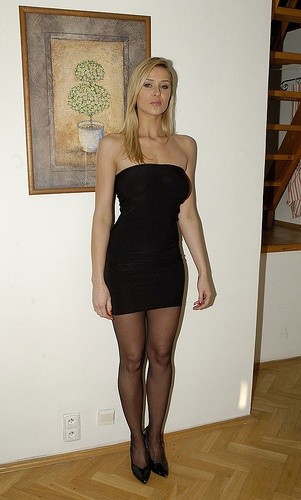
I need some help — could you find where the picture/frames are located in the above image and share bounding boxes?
[18,5,152,195]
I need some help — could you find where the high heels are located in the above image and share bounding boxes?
[144,425,169,478]
[130,440,151,484]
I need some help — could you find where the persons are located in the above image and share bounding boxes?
[90,56,216,484]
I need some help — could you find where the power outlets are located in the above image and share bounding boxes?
[63,412,81,442]
